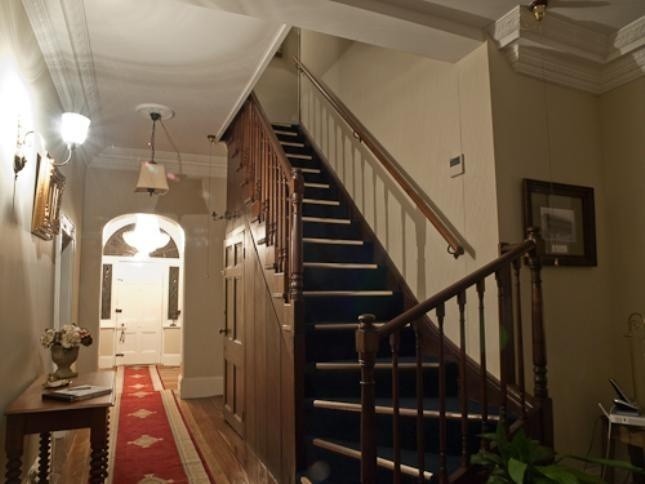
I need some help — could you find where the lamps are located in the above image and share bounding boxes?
[134,113,170,197]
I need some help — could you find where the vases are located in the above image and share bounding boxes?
[52,344,79,379]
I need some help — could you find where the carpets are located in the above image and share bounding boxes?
[106,364,215,484]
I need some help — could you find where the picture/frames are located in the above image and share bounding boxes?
[31,153,67,241]
[523,178,597,266]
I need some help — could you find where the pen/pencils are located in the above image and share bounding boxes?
[68,387,91,391]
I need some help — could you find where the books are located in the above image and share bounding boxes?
[42,384,114,403]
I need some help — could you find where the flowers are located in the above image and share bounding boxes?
[40,323,93,350]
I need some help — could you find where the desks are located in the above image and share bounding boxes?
[3,370,116,483]
